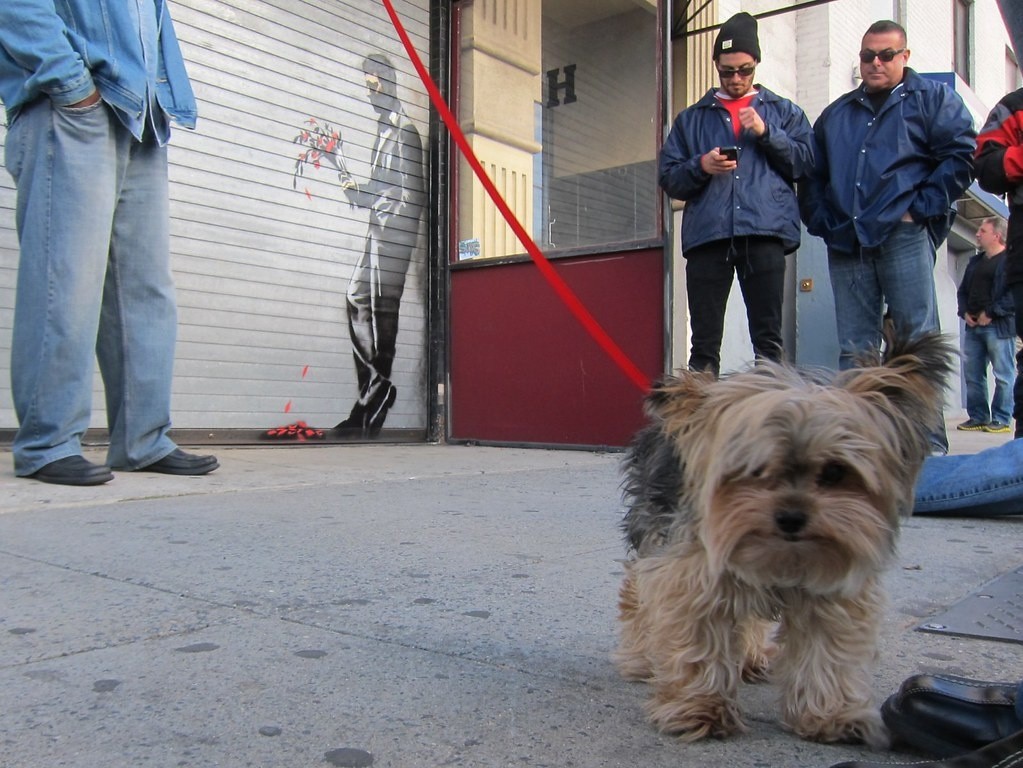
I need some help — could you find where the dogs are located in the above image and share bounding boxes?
[616,317,965,748]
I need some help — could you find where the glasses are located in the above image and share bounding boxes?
[858,49,903,63]
[715,62,754,78]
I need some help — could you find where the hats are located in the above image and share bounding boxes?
[712,12,761,63]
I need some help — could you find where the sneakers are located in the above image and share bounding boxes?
[981,421,1010,432]
[957,418,988,432]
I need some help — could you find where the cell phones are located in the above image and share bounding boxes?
[719,146,738,168]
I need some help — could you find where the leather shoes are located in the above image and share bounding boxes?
[830,731,1023,768]
[879,674,1023,753]
[143,446,219,475]
[29,455,115,484]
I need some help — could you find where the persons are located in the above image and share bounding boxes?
[658,11,819,381]
[974,86,1023,440]
[881,673,1023,758]
[0,0,221,485]
[797,20,978,455]
[957,215,1016,432]
[911,436,1023,516]
